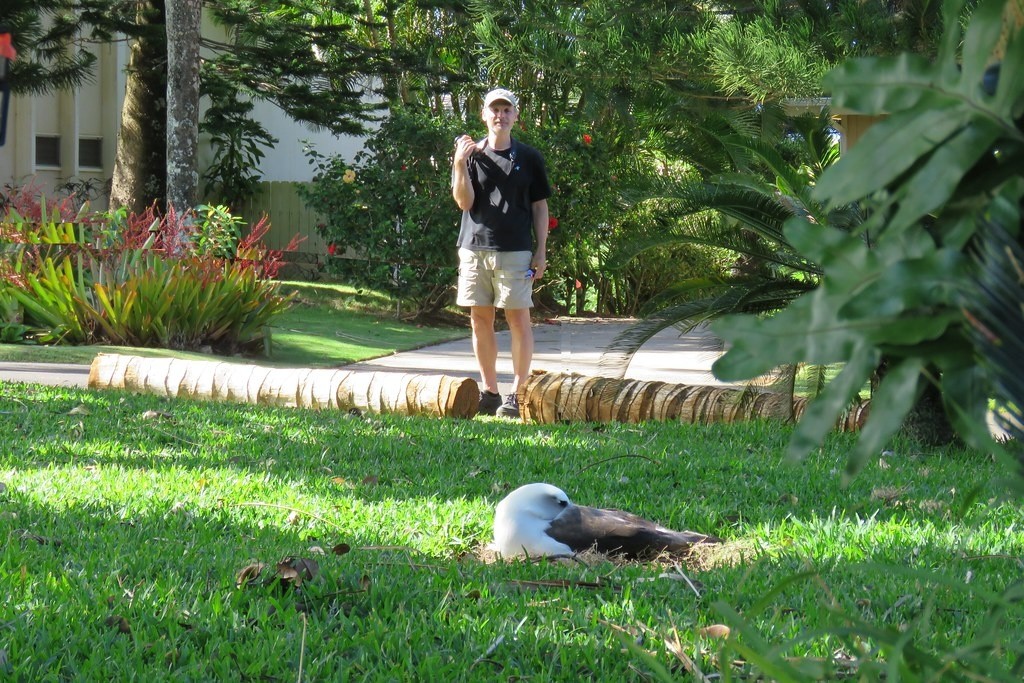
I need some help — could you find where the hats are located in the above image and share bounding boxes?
[484,89,516,109]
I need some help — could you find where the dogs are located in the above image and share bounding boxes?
[488,483,708,559]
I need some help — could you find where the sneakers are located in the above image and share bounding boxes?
[496,392,520,418]
[476,390,502,416]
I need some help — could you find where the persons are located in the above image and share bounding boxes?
[452,89,553,417]
